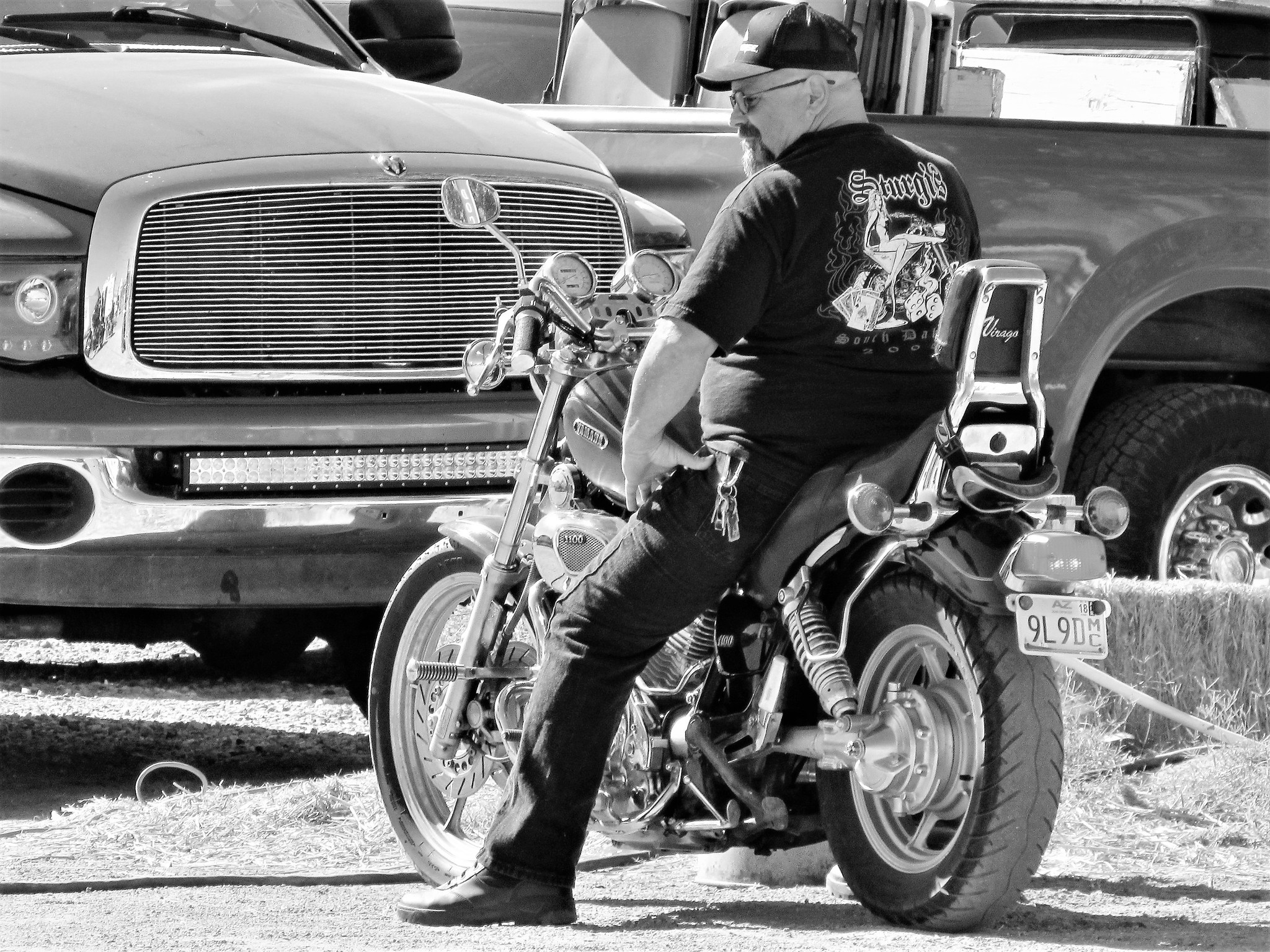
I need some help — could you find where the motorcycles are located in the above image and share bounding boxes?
[364,175,1132,938]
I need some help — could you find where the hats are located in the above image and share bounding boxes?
[695,2,859,91]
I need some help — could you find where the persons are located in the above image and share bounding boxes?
[394,1,982,925]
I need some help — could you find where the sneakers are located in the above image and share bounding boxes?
[398,861,578,927]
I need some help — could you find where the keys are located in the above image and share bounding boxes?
[710,482,740,541]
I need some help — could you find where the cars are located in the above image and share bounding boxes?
[0,0,1270,669]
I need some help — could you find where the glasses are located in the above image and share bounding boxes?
[730,79,835,114]
